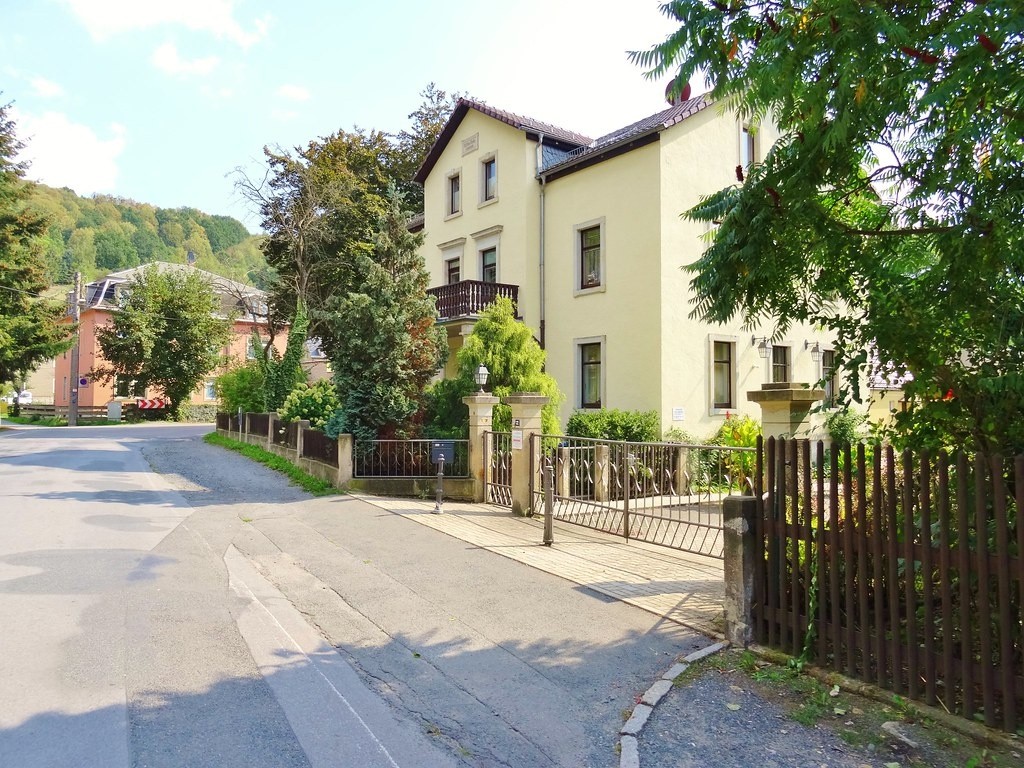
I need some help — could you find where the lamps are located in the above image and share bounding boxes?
[752,335,773,359]
[475,363,491,392]
[805,340,824,362]
